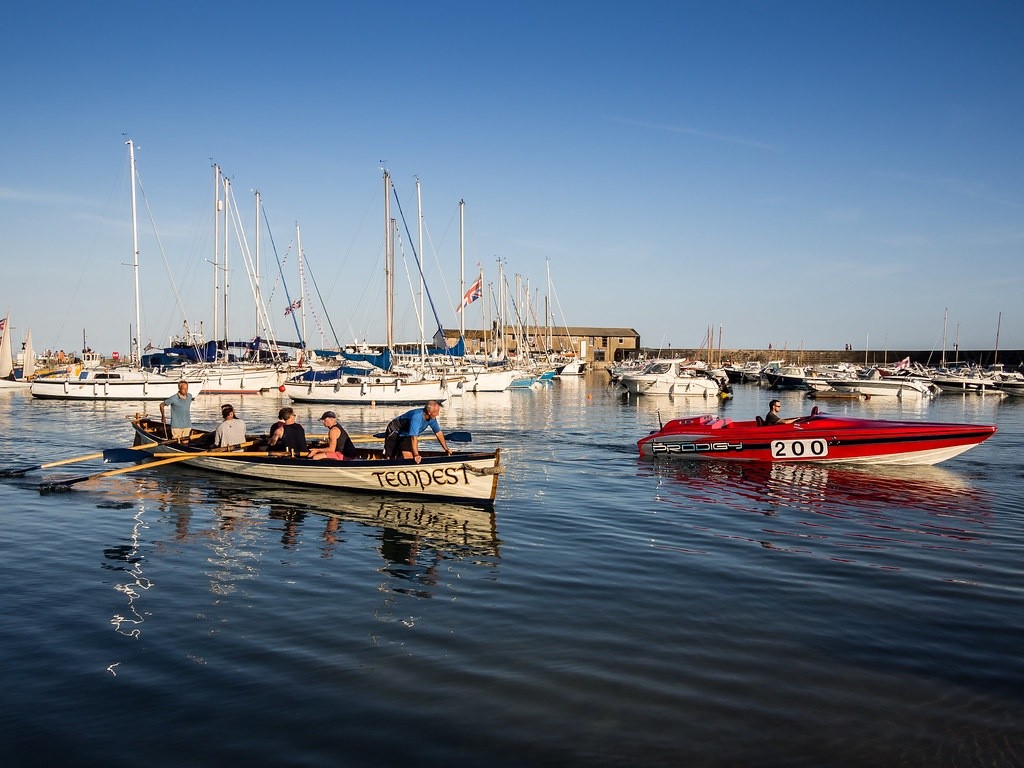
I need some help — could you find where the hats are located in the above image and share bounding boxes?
[320,411,336,421]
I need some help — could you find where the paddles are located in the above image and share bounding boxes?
[102,448,311,463]
[13,431,216,474]
[61,437,270,486]
[263,430,385,438]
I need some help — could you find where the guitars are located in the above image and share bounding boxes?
[351,432,473,443]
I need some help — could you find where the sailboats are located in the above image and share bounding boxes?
[0,160,1024,399]
[31,139,208,401]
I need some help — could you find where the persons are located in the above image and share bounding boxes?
[765,400,801,426]
[308,411,357,460]
[383,402,452,464]
[257,407,309,452]
[160,381,192,445]
[200,404,246,452]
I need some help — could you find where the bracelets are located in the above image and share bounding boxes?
[414,455,420,456]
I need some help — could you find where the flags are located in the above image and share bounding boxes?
[284,300,301,316]
[457,276,482,312]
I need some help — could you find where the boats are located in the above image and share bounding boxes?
[636,412,999,467]
[125,411,503,502]
[638,454,978,506]
[135,455,503,557]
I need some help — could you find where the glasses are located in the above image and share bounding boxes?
[776,406,780,408]
[290,414,296,416]
